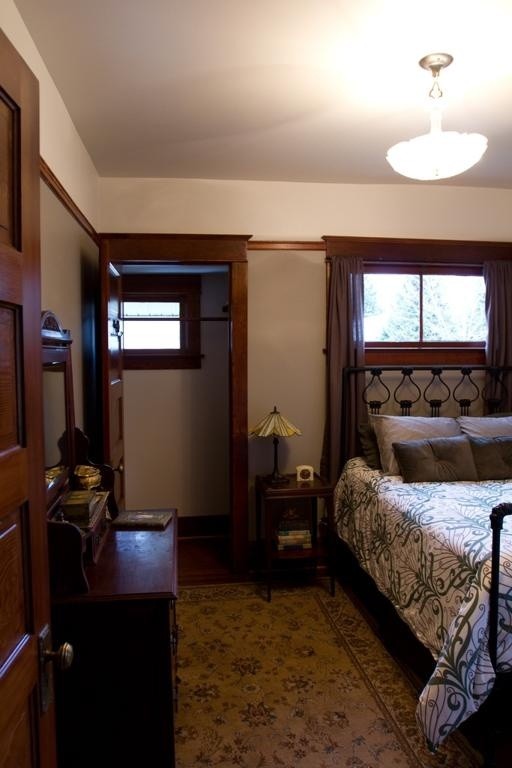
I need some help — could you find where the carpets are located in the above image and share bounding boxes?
[171,577,484,768]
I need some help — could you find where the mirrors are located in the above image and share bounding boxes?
[41,310,75,508]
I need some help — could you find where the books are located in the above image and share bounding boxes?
[113,510,173,531]
[273,521,313,551]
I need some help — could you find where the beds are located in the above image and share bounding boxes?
[335,364,512,756]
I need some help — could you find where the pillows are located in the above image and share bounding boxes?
[457,416,512,437]
[468,434,512,481]
[392,434,478,483]
[368,413,460,477]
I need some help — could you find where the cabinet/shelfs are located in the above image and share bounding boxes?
[50,509,177,768]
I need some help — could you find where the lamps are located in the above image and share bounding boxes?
[249,406,302,483]
[385,52,489,180]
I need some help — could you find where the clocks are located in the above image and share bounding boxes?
[296,464,313,481]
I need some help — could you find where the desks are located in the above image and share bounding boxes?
[257,472,335,602]
